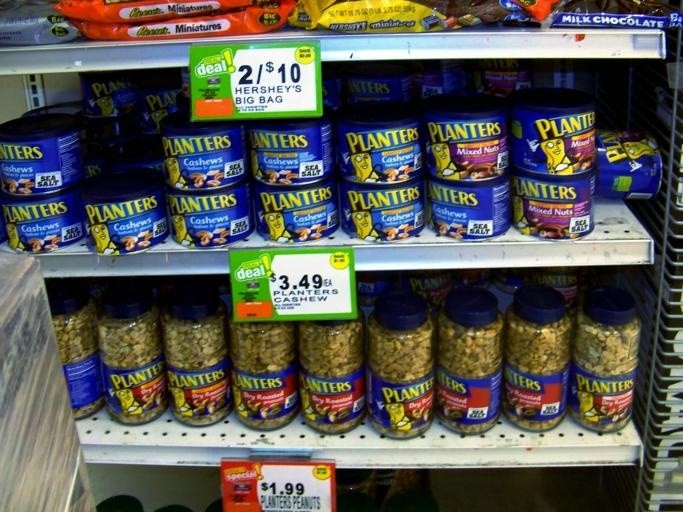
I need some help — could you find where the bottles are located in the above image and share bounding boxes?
[43,286,643,440]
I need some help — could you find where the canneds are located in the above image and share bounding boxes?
[0,60,663,255]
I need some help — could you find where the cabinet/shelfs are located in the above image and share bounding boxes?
[0,28,667,469]
